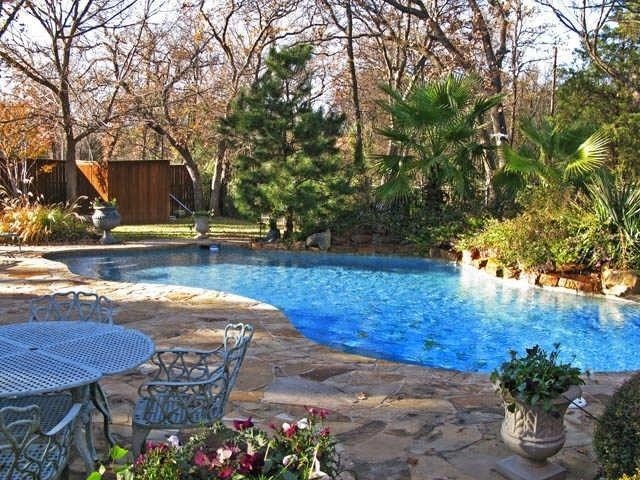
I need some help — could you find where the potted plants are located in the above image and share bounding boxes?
[92,197,122,244]
[191,209,214,239]
[453,216,548,285]
[490,343,598,480]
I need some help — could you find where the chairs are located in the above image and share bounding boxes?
[0,395,82,480]
[29,292,113,460]
[133,323,253,460]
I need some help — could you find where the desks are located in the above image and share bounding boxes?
[0,321,155,475]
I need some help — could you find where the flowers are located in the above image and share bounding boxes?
[86,407,341,480]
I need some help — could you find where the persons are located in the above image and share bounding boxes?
[259,216,293,242]
